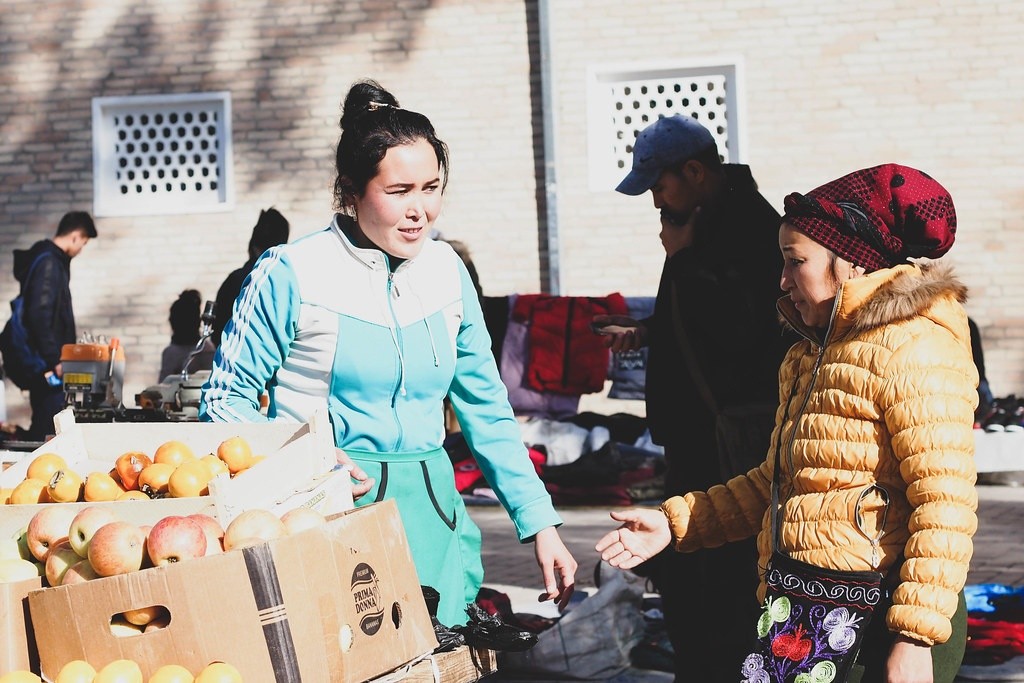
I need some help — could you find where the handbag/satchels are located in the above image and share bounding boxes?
[739,549,884,683]
[0,252,59,389]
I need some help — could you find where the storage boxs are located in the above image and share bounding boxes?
[0,416,498,683]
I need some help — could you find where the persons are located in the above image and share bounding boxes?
[588,116,805,682]
[211,208,290,348]
[159,289,216,383]
[13,211,97,440]
[432,229,482,435]
[197,79,577,642]
[595,162,979,683]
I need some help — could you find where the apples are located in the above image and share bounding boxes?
[0,508,328,683]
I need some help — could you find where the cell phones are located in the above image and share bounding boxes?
[589,322,635,336]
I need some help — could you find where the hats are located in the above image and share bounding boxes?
[782,164,957,271]
[615,116,717,195]
